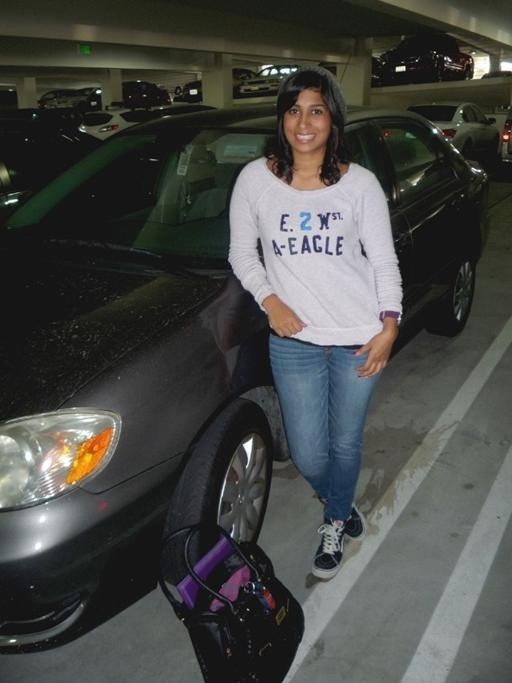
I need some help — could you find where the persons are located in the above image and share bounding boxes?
[228,65,403,579]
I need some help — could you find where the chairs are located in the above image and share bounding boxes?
[185,162,238,224]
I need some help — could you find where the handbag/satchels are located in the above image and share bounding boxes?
[156,521,305,683]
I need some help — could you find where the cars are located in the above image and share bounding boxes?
[38,80,171,110]
[379,36,475,87]
[182,63,312,97]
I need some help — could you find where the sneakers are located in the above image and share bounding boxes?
[311,496,367,579]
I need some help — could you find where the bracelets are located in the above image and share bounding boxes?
[380,311,401,325]
